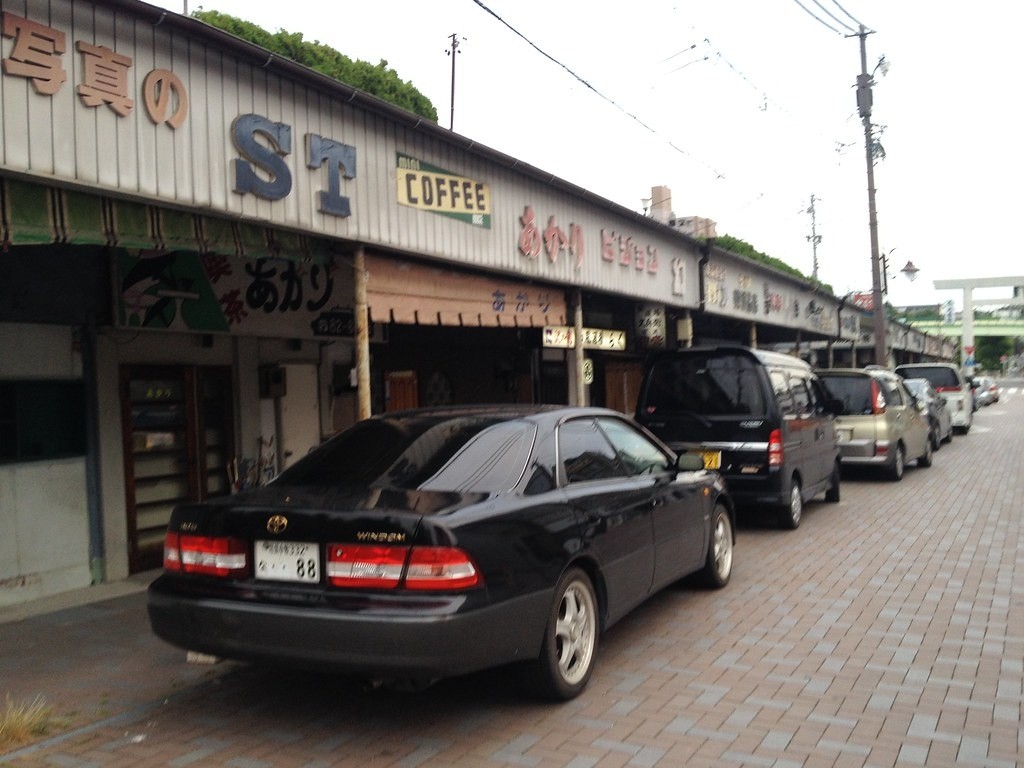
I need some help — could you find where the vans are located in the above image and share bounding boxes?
[634,346,848,529]
[895,364,975,433]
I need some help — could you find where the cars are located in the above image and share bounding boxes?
[904,378,953,450]
[815,365,934,481]
[965,375,998,412]
[147,402,735,702]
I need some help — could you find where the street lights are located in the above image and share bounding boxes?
[871,261,921,369]
